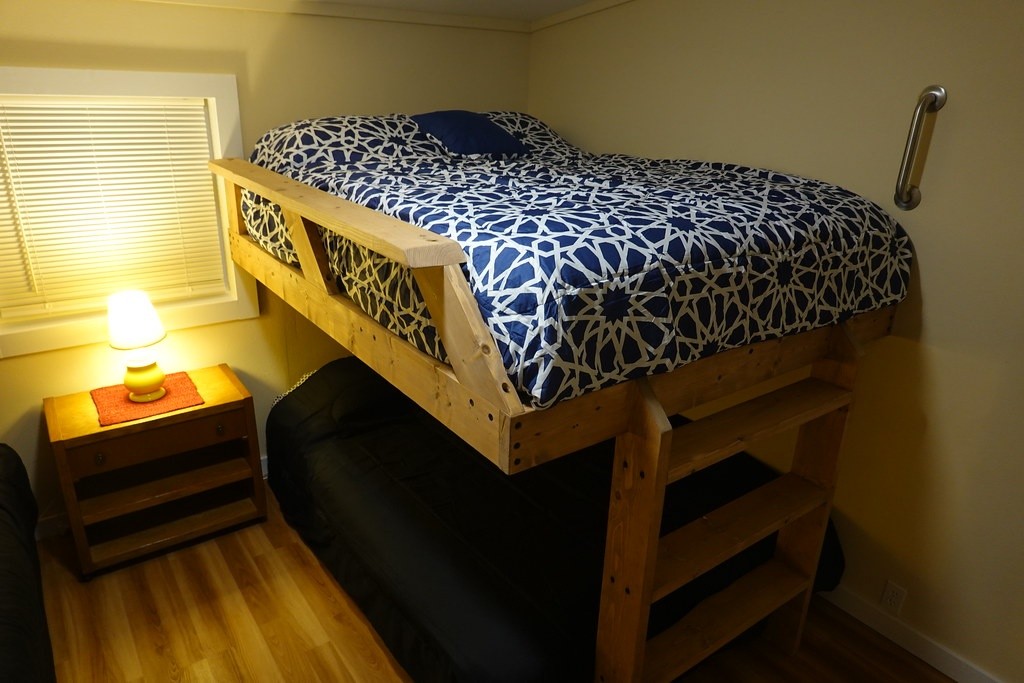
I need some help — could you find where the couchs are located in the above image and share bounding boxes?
[0,442,57,683]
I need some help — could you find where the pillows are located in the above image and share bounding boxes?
[410,110,533,160]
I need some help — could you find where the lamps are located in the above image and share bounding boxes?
[108,288,168,404]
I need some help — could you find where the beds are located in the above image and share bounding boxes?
[208,108,913,682]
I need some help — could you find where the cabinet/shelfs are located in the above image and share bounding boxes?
[43,362,267,582]
